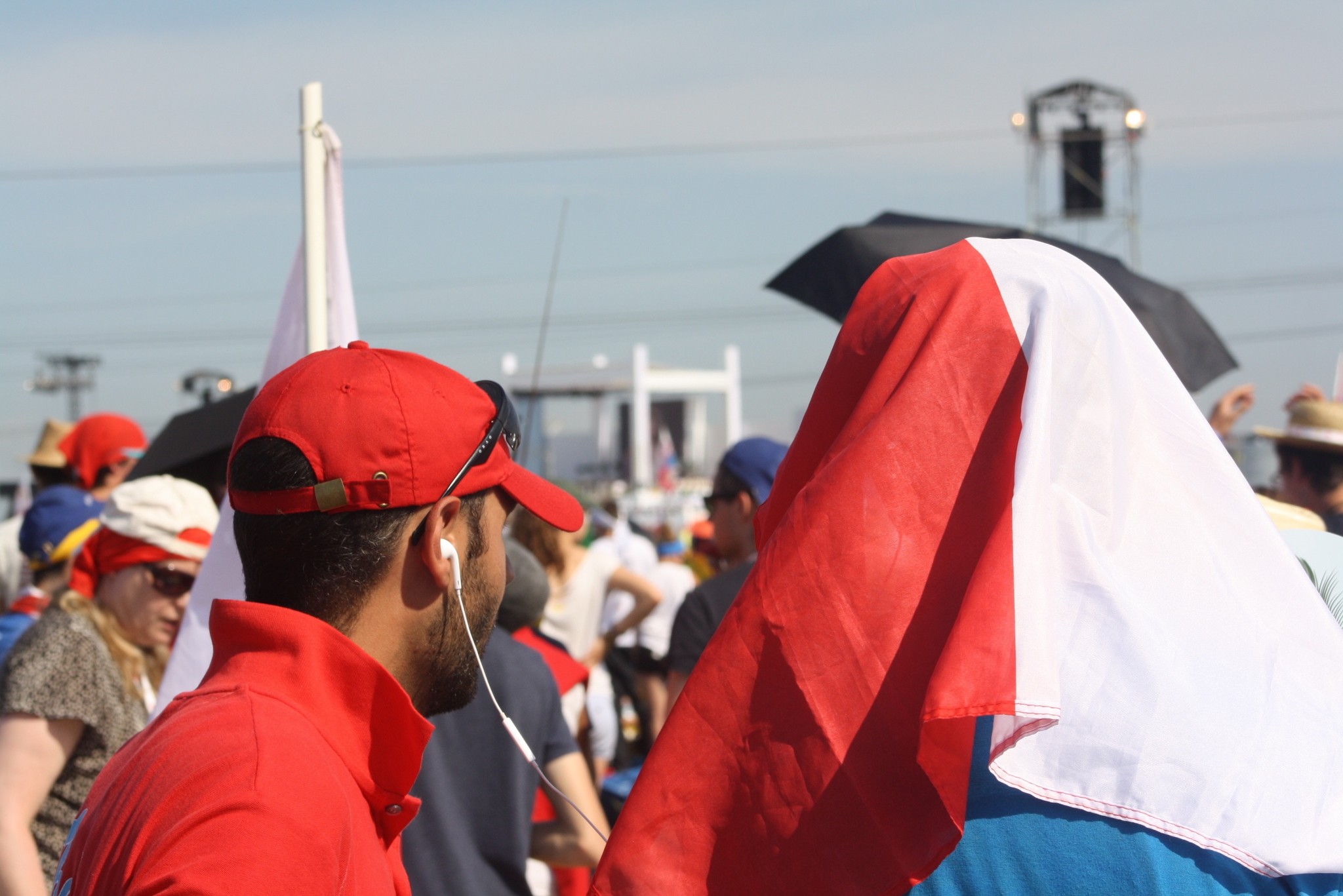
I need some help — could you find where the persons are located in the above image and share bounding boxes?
[0,475,221,896]
[586,236,1343,895]
[53,342,584,896]
[401,627,612,896]
[492,501,720,896]
[0,414,149,672]
[669,436,788,714]
[1208,385,1343,630]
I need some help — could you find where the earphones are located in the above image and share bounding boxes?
[440,538,461,589]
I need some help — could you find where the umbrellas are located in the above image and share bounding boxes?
[767,212,1238,390]
[125,383,257,482]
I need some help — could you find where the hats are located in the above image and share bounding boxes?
[1253,402,1343,454]
[227,341,584,533]
[25,420,73,470]
[723,438,790,505]
[99,472,220,560]
[19,486,109,569]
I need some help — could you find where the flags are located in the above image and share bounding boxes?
[152,128,358,718]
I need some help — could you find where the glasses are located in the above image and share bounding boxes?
[143,563,195,597]
[410,380,521,545]
[1274,439,1343,492]
[705,490,736,515]
